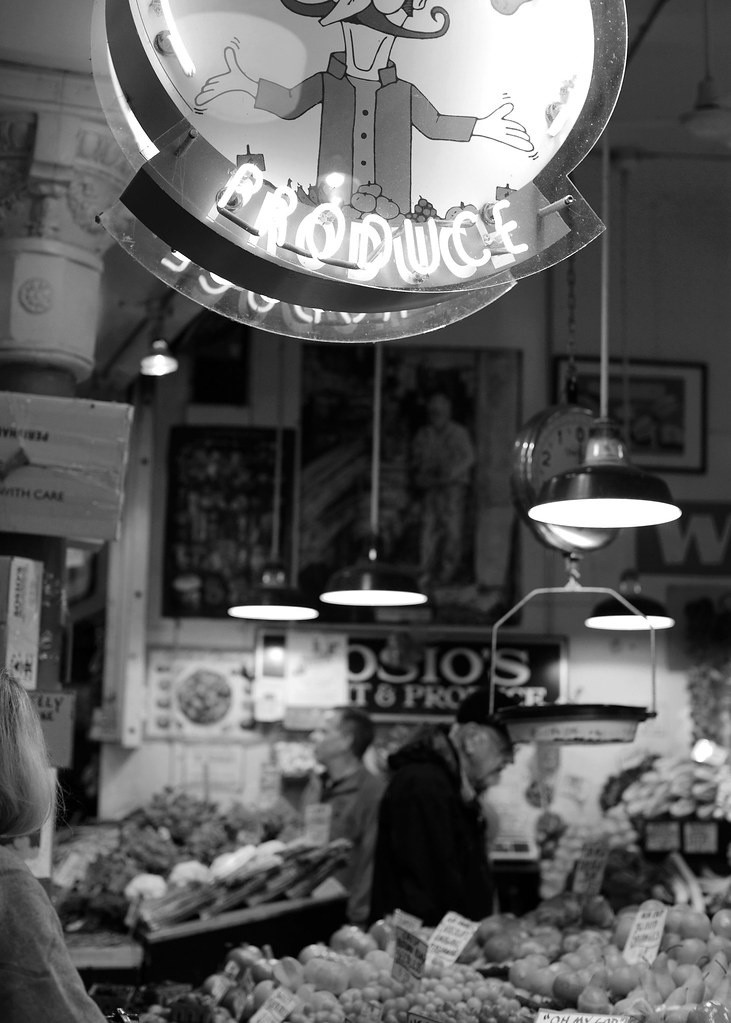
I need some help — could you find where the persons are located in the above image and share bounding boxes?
[369,690,514,930]
[0,672,108,1023]
[311,707,384,932]
[418,397,471,589]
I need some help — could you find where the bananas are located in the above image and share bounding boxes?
[622,753,731,824]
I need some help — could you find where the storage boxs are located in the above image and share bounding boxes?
[251,628,352,722]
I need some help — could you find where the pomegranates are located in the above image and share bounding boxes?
[202,920,400,1023]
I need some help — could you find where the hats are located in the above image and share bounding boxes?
[456,688,519,745]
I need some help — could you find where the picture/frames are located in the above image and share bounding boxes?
[551,353,710,476]
[295,339,525,624]
[161,422,297,619]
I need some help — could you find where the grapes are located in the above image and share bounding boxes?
[293,965,540,1023]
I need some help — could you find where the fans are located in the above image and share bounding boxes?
[679,0,731,137]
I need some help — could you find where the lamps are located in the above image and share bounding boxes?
[315,338,430,611]
[583,569,675,632]
[526,136,684,531]
[225,338,321,622]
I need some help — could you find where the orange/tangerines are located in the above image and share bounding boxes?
[460,898,731,997]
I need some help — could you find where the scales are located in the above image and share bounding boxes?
[489,357,659,745]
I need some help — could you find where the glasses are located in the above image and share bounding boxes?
[485,729,521,757]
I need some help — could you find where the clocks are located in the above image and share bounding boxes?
[510,407,626,555]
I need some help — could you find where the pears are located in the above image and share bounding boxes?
[574,946,731,1023]
